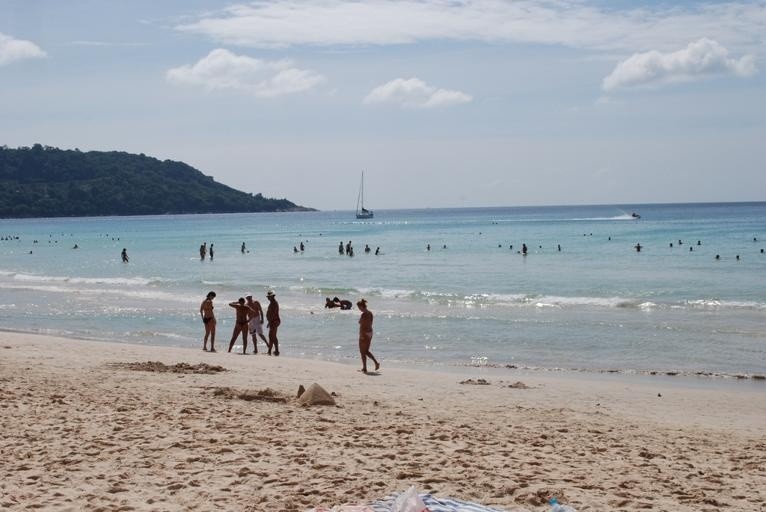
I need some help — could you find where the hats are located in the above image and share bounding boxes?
[266,289,276,297]
[244,291,253,297]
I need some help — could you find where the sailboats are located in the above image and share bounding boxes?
[356,170,373,219]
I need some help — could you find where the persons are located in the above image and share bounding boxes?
[325,297,345,309]
[199,290,218,352]
[120,248,129,263]
[356,299,380,372]
[244,293,269,354]
[523,243,528,254]
[635,243,642,252]
[265,289,281,356]
[195,237,381,263]
[227,297,257,355]
[333,296,353,310]
